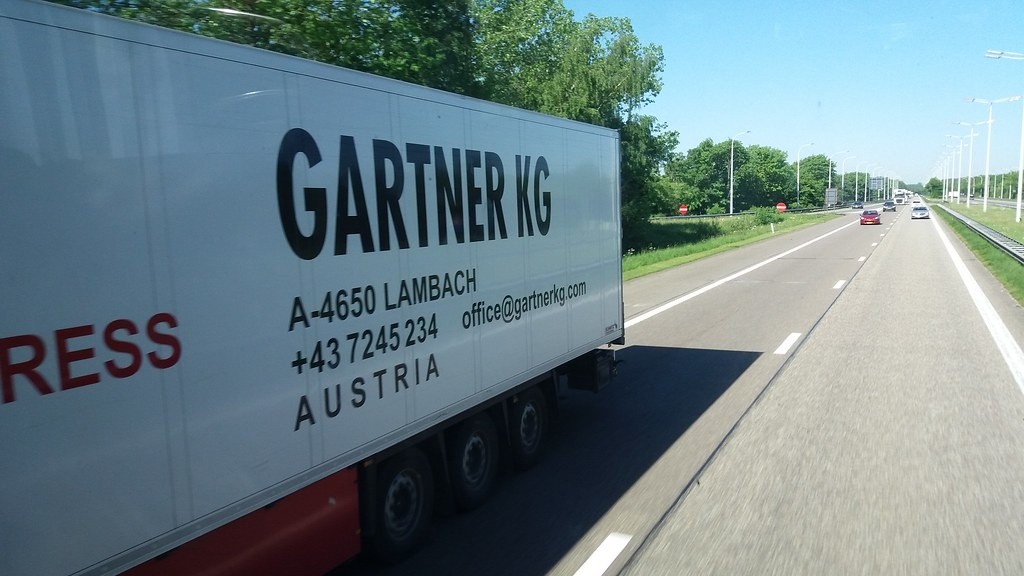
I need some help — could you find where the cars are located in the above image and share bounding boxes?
[852,201,864,209]
[911,206,930,219]
[913,197,921,202]
[860,210,882,224]
[882,202,899,211]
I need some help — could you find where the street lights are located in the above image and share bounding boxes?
[969,96,1021,209]
[730,130,750,216]
[829,150,844,188]
[954,120,992,206]
[797,143,814,207]
[937,132,978,203]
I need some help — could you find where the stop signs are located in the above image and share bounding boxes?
[777,202,787,212]
[679,205,688,215]
